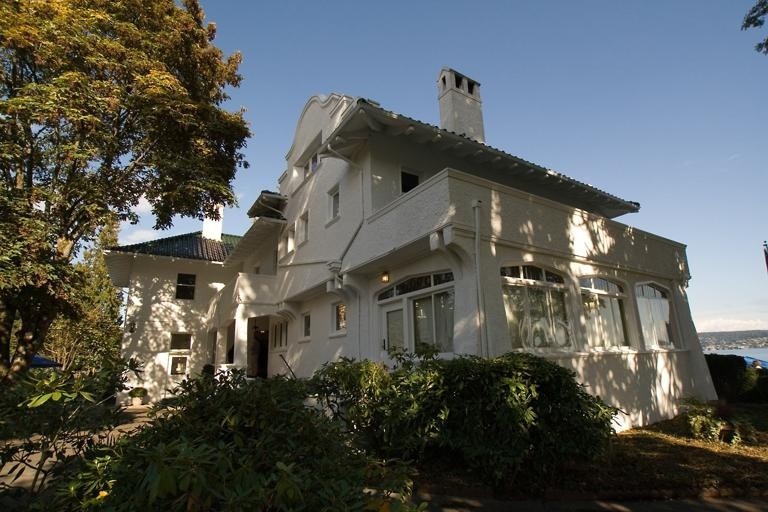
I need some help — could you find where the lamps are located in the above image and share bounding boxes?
[381,270,389,283]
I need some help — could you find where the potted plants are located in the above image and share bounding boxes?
[128,387,148,406]
